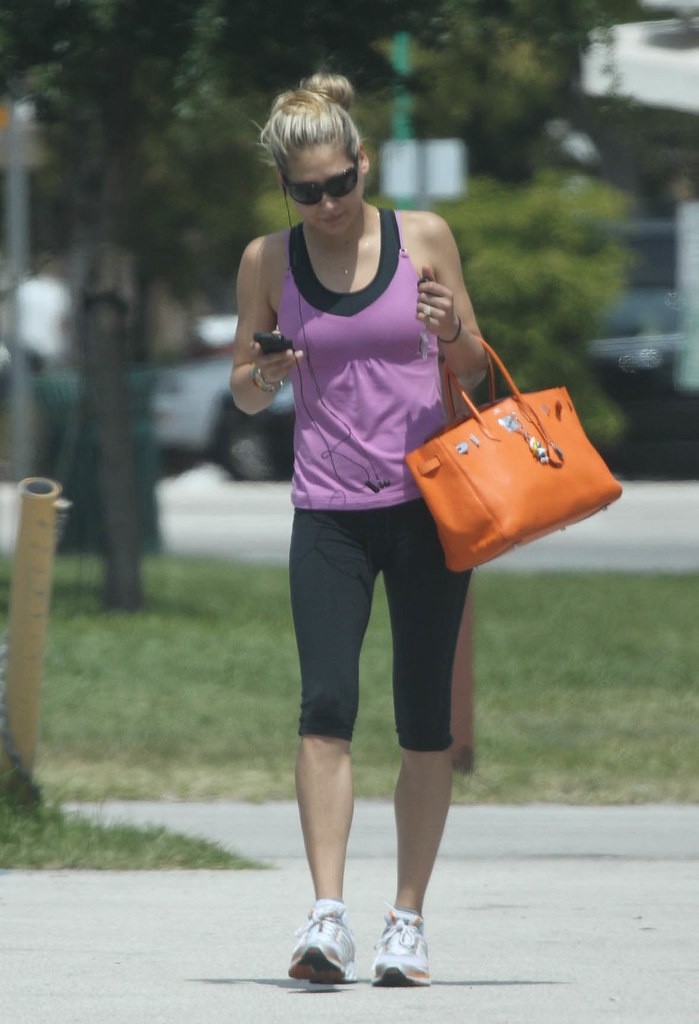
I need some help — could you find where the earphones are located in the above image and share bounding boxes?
[282,181,288,192]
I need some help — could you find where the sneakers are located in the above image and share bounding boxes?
[371,907,432,988]
[287,907,358,983]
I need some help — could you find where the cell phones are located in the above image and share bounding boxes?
[253,334,294,355]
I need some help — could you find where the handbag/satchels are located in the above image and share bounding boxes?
[401,338,623,572]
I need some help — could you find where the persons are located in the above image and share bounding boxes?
[230,71,490,989]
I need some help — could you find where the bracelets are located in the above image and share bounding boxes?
[251,363,287,394]
[437,316,461,344]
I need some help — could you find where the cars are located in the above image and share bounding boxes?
[150,312,298,483]
[585,332,699,482]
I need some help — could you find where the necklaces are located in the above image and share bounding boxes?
[306,229,373,274]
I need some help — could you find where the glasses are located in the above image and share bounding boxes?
[284,156,363,209]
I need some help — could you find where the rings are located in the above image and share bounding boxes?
[424,304,431,319]
